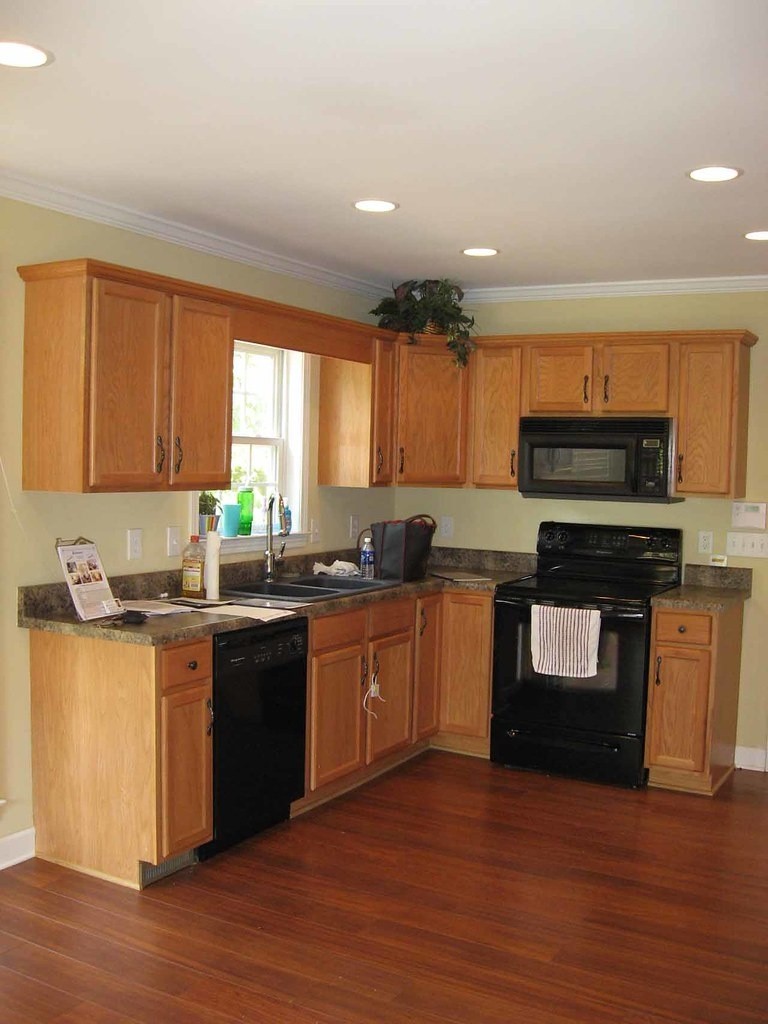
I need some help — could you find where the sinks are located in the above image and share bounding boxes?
[224,584,339,598]
[287,576,387,590]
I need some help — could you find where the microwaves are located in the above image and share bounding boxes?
[518,413,686,505]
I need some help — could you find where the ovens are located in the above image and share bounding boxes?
[489,600,652,792]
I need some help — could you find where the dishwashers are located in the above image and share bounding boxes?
[195,616,309,863]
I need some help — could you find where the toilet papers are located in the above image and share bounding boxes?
[203,530,222,600]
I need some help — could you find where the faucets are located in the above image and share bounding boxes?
[264,492,288,584]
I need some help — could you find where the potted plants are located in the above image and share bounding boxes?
[198,489,223,539]
[368,277,480,369]
[232,463,268,536]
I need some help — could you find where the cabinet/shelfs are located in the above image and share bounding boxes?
[305,594,411,808]
[644,605,744,800]
[523,331,680,417]
[28,629,212,890]
[411,588,438,758]
[437,586,496,760]
[316,324,400,490]
[16,257,235,495]
[395,332,468,489]
[675,329,759,500]
[468,335,525,491]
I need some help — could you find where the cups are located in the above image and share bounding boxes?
[222,504,241,537]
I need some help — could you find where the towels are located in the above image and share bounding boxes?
[312,558,363,578]
[530,604,602,679]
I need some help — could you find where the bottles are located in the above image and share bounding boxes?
[361,538,374,580]
[236,486,254,535]
[181,535,206,600]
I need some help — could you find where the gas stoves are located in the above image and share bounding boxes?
[494,521,683,611]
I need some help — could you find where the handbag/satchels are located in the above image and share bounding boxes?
[357,512,437,582]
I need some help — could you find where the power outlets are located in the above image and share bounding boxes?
[697,529,713,555]
[127,528,144,561]
[439,515,453,539]
[310,518,322,544]
[349,513,360,539]
